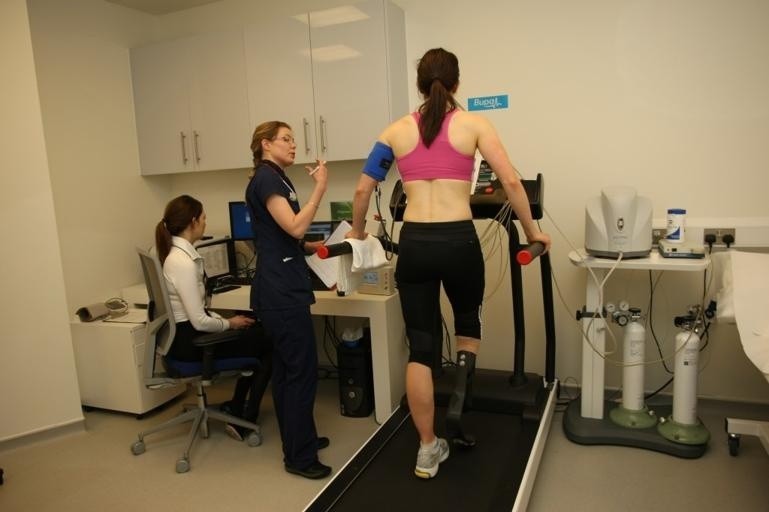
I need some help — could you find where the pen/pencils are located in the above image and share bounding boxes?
[309,160,327,176]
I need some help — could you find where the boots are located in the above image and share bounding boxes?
[444,351,478,446]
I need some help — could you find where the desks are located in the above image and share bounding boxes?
[123,279,411,425]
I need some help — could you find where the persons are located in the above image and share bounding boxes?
[155,195,272,439]
[345,48,550,480]
[244,121,332,477]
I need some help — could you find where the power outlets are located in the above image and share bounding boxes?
[704,228,735,245]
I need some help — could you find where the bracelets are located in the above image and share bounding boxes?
[308,198,321,209]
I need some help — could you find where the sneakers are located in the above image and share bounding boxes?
[415,437,449,478]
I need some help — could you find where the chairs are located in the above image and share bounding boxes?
[131,246,263,473]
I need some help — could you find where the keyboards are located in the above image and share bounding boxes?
[223,277,250,285]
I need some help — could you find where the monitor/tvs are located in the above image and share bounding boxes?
[227,200,261,241]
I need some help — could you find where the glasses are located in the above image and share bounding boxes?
[273,133,296,144]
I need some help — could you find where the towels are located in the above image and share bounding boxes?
[336,234,389,295]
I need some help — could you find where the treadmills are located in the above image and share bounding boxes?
[303,173,562,512]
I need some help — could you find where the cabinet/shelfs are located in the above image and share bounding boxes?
[68,309,187,419]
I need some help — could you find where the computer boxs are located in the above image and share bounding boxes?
[335,326,375,418]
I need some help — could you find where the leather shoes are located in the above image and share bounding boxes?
[318,436,329,450]
[285,459,331,480]
[221,399,257,440]
